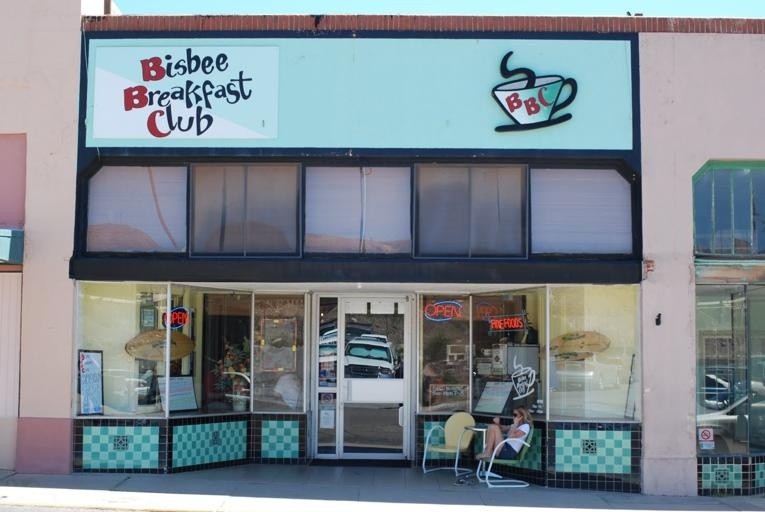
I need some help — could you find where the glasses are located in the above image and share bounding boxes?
[512,413,517,417]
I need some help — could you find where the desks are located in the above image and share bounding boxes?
[464,425,491,481]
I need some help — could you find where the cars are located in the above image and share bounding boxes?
[320,329,398,378]
[555,351,637,392]
[98,366,151,408]
[696,363,765,459]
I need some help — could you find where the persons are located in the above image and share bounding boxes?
[476,406,532,459]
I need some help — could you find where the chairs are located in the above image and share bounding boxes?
[422,412,477,477]
[476,422,534,488]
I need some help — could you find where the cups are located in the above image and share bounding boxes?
[480,348,492,357]
[491,75,577,124]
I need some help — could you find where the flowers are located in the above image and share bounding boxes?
[209,336,251,403]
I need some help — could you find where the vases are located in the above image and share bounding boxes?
[233,375,247,412]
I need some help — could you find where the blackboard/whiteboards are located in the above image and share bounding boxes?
[157,375,199,412]
[474,381,514,414]
[78,348,103,416]
